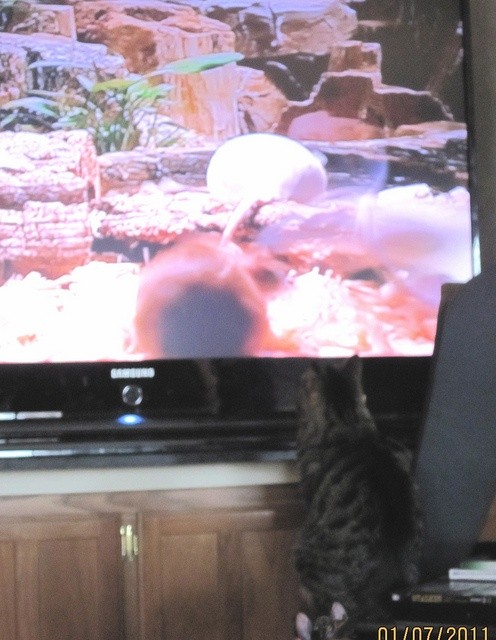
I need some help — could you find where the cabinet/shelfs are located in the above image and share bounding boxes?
[142,487,312,640]
[1,496,141,640]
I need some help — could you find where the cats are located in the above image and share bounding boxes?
[280,352,426,618]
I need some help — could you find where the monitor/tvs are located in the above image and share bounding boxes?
[0,3,484,473]
